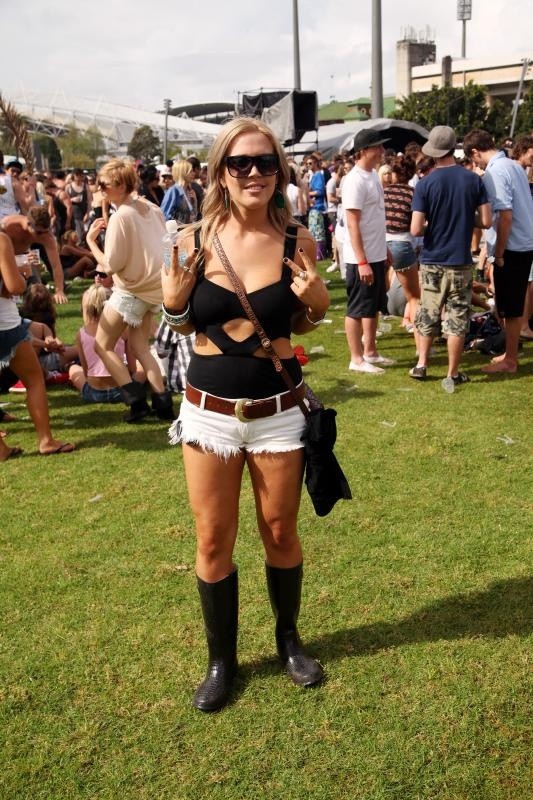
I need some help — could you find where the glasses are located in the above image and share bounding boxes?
[93,270,108,278]
[98,180,112,189]
[306,162,314,165]
[32,226,49,235]
[224,154,278,177]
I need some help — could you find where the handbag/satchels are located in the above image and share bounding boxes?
[299,408,352,516]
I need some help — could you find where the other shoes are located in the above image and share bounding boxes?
[47,371,69,384]
[8,381,27,391]
[326,264,339,274]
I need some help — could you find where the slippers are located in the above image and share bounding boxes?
[10,447,21,457]
[56,442,71,453]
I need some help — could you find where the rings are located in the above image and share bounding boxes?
[183,265,190,272]
[298,270,307,279]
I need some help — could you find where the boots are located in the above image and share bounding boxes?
[193,564,238,711]
[264,557,323,685]
[119,378,150,422]
[150,391,174,418]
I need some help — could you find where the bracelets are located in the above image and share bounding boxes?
[306,311,326,326]
[358,260,368,265]
[161,302,191,326]
[495,257,504,258]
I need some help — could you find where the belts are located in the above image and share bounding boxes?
[185,380,305,422]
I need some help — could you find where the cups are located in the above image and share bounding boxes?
[15,254,27,266]
[30,249,40,266]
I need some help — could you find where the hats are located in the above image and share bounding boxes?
[422,126,456,157]
[354,129,392,151]
[161,170,173,178]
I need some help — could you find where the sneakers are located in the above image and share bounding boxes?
[448,372,471,385]
[366,355,394,364]
[350,360,384,374]
[409,365,426,379]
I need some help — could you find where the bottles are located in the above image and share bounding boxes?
[162,220,188,275]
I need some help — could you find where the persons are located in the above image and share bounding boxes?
[0,126,533,461]
[86,157,177,424]
[160,114,330,713]
[341,128,398,373]
